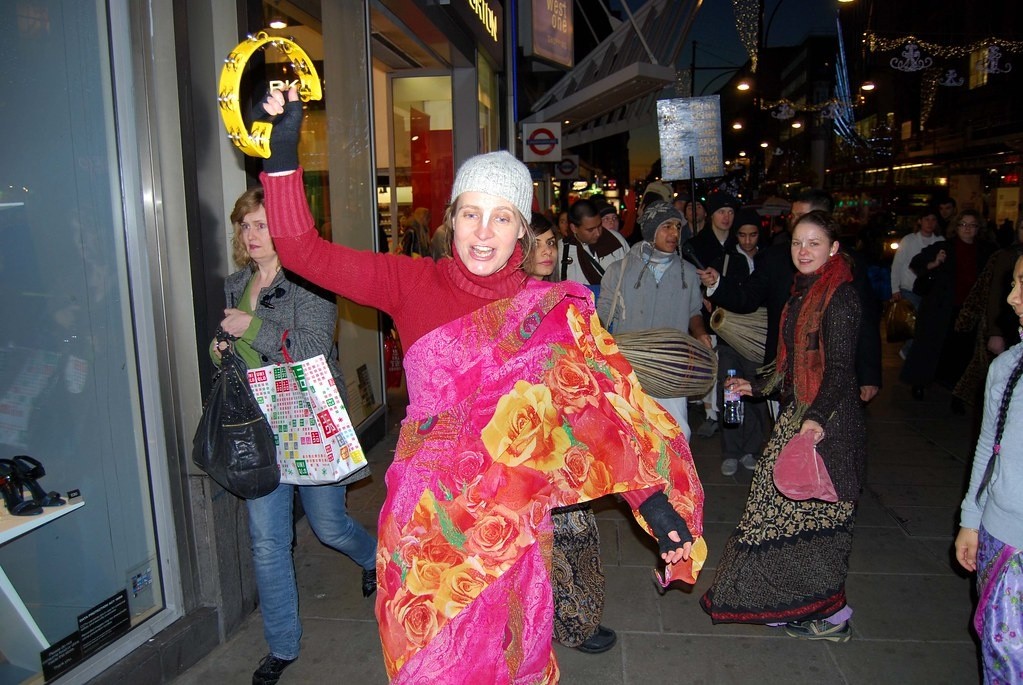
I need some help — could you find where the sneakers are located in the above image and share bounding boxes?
[361,569,377,600]
[784,617,853,644]
[252,653,299,684]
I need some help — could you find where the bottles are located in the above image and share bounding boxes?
[724,369,741,423]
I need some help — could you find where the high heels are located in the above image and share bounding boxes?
[0,455,67,516]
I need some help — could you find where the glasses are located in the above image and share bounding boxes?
[959,221,979,229]
[601,215,619,222]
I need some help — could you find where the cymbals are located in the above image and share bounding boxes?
[217,31,323,159]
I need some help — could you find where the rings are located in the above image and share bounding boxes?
[815,435,823,437]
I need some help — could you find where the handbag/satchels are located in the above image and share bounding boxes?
[190,320,282,499]
[243,335,369,486]
[884,298,919,344]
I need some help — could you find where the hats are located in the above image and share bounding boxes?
[634,200,689,291]
[590,199,617,216]
[707,191,736,215]
[734,207,762,230]
[641,181,675,204]
[450,149,534,225]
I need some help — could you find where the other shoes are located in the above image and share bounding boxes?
[721,454,757,476]
[912,383,924,401]
[698,417,719,437]
[576,624,616,654]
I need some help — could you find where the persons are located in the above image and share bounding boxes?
[955,253,1023,685]
[520,191,792,654]
[890,196,1023,408]
[699,211,866,643]
[378,207,453,339]
[209,187,377,685]
[252,80,692,685]
[696,189,882,511]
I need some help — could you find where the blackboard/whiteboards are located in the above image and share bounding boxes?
[656,95,725,181]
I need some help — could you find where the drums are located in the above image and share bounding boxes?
[611,328,718,399]
[709,306,768,364]
